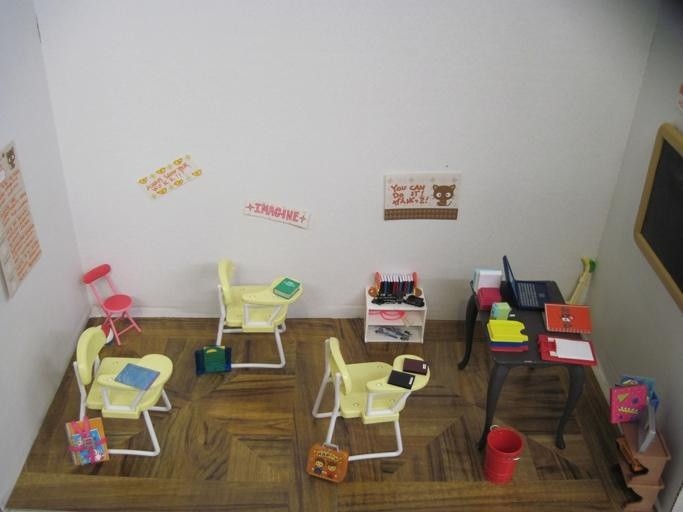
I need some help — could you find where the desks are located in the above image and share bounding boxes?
[241,276,303,332]
[361,354,430,424]
[97,354,173,419]
[457,280,586,454]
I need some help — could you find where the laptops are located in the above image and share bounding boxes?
[503,255,551,312]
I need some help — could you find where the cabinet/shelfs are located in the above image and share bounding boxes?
[364,287,427,344]
[616,421,672,512]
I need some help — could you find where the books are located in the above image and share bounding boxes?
[65,417,110,467]
[273,278,300,300]
[378,272,414,295]
[542,303,592,334]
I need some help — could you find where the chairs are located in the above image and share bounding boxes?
[82,264,142,346]
[72,327,173,457]
[215,257,303,369]
[312,337,430,462]
